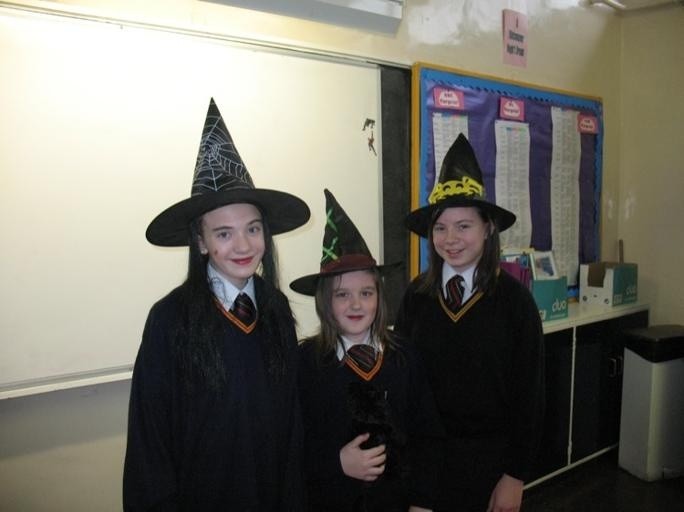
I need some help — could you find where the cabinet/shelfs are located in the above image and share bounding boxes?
[517,296,650,490]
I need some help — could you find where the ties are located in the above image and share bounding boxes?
[349,343,377,373]
[235,294,252,323]
[446,274,465,315]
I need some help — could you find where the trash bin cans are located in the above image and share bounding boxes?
[616,323,684,483]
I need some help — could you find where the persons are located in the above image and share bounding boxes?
[294,189,436,511]
[123,96,313,512]
[391,132,547,511]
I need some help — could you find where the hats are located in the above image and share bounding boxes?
[145,97,311,248]
[404,133,516,238]
[289,189,403,296]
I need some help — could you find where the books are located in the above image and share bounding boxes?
[498,245,558,291]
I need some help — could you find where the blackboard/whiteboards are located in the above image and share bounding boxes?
[0,0,387,402]
